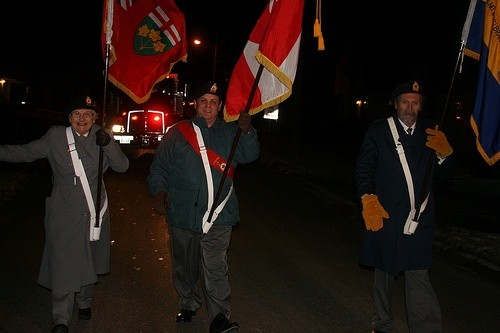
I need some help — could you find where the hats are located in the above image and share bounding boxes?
[69,95,97,115]
[194,79,221,100]
[390,79,427,100]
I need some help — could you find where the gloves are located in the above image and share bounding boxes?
[152,192,167,217]
[95,129,112,147]
[237,109,252,130]
[361,194,389,231]
[425,128,453,159]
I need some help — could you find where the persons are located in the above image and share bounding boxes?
[148,82,260,333]
[356,81,457,333]
[0,95,129,333]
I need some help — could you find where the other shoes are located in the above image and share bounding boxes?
[176,309,193,322]
[51,324,68,333]
[209,313,241,333]
[79,307,92,321]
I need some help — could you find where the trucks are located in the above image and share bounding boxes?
[107,74,198,146]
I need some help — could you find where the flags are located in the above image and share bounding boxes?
[101,0,189,103]
[459,0,500,166]
[222,0,325,122]
